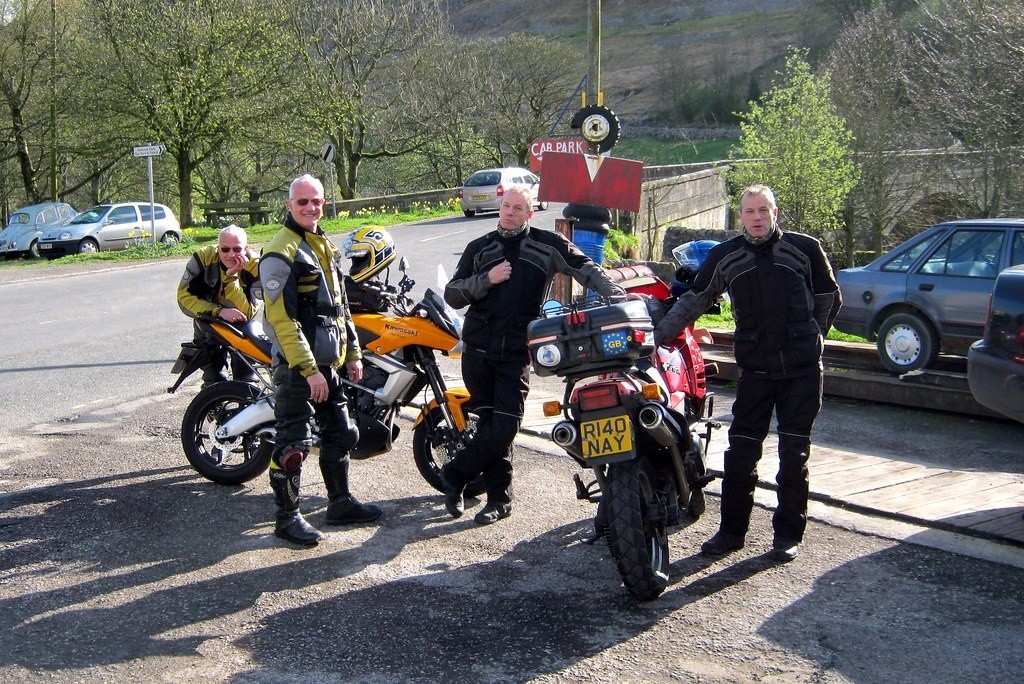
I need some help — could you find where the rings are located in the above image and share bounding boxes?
[316,391,318,393]
[241,265,244,267]
[233,317,235,320]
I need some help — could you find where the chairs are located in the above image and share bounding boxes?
[488,175,498,182]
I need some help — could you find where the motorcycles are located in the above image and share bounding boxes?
[525,273,724,601]
[165,225,516,498]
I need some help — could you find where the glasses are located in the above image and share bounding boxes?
[291,199,324,206]
[219,246,244,252]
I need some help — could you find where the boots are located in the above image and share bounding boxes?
[268,468,324,544]
[319,456,383,523]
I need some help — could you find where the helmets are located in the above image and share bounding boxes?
[344,226,396,283]
[672,240,722,296]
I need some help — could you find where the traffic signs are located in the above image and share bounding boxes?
[133,145,163,157]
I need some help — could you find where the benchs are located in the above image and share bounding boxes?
[919,261,997,278]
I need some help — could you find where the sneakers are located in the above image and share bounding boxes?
[439,466,463,517]
[701,531,745,554]
[773,536,799,559]
[475,505,509,523]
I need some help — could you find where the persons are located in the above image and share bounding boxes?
[177,224,262,452]
[440,185,628,524]
[258,174,382,545]
[655,185,842,562]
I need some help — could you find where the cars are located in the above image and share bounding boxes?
[458,166,550,219]
[966,262,1023,425]
[0,201,100,259]
[832,215,1024,377]
[35,201,183,261]
